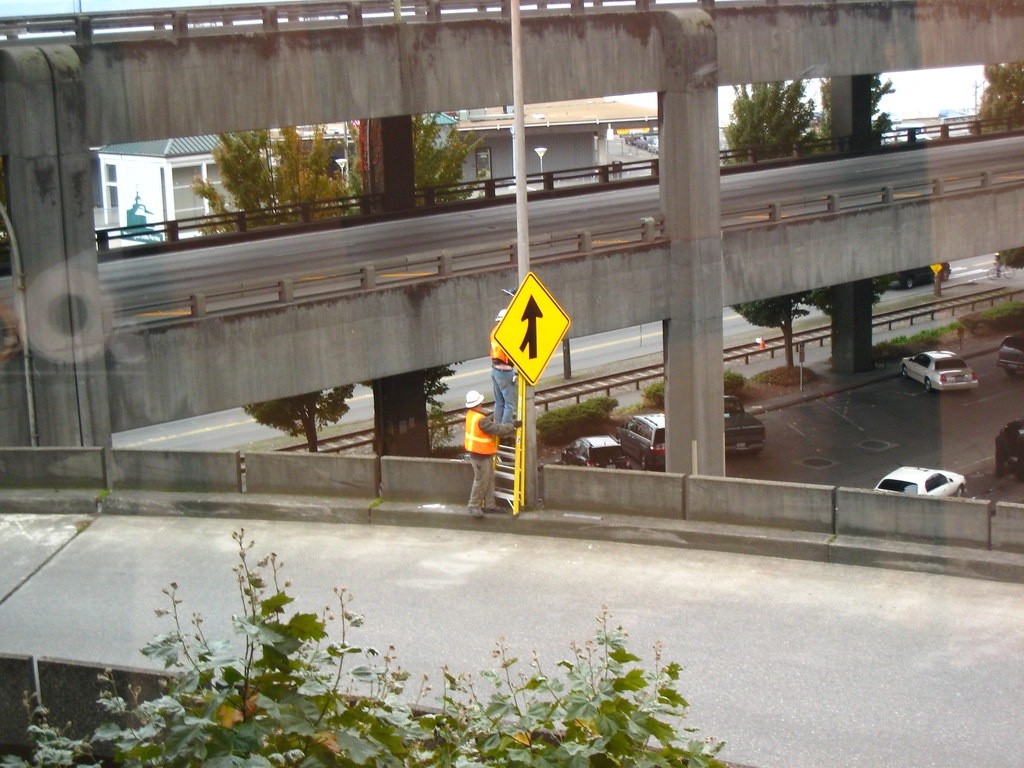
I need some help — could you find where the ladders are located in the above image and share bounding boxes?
[478,347,525,520]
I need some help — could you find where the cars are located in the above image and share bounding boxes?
[901,350,977,393]
[873,465,969,499]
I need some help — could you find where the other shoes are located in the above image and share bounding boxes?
[468,505,484,518]
[481,506,508,513]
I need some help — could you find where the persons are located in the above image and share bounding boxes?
[490,309,517,438]
[464,390,515,517]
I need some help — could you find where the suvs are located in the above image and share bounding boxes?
[562,433,632,471]
[995,333,1024,376]
[724,397,766,458]
[994,421,1024,481]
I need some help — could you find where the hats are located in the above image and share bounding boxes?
[465,390,484,408]
[495,309,506,321]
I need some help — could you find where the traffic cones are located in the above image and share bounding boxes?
[759,336,766,349]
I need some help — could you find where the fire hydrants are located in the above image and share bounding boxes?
[993,252,1003,277]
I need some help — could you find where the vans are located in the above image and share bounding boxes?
[895,262,951,289]
[615,413,665,471]
